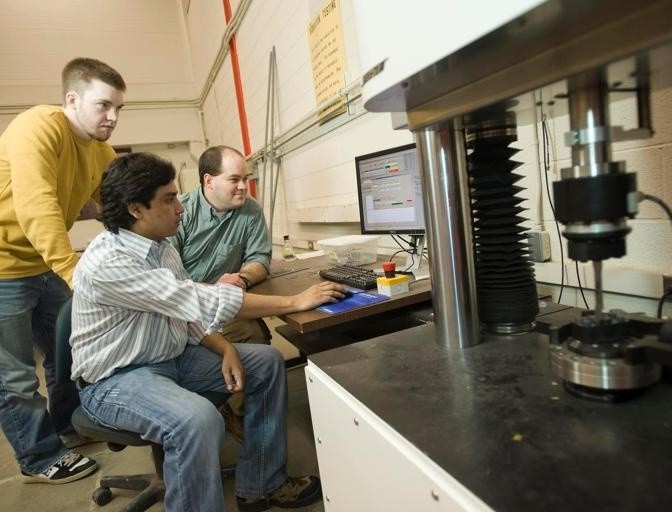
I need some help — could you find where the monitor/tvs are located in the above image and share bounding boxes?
[354,143,431,284]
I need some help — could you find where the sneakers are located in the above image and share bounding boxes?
[235,475,320,512]
[21,451,97,485]
[60,431,105,448]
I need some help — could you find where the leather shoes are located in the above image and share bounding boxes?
[220,404,244,444]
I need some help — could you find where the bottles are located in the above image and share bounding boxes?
[283,235,296,261]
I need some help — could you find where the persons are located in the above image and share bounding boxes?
[0,56,127,485]
[68,151,348,512]
[165,142,274,446]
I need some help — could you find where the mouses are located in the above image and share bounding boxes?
[319,290,354,305]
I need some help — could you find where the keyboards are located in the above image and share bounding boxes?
[319,263,383,291]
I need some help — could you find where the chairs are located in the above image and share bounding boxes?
[53,296,237,512]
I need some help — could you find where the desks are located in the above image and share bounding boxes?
[249,241,433,335]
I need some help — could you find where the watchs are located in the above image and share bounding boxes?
[239,275,252,290]
